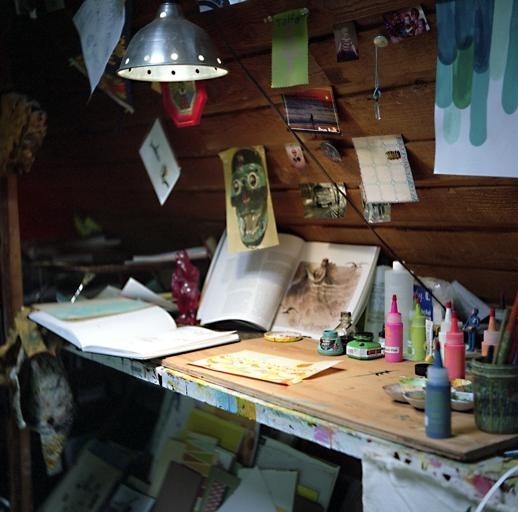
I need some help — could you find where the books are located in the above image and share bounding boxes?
[354,133,418,207]
[39,390,340,512]
[198,225,380,342]
[27,302,241,360]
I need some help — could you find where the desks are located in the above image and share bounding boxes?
[62,297,515,505]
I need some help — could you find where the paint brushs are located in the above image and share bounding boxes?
[70,272,94,303]
[492,300,516,366]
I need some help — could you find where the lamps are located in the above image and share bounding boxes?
[116,3,230,84]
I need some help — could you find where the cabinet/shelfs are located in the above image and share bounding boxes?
[1,161,36,512]
